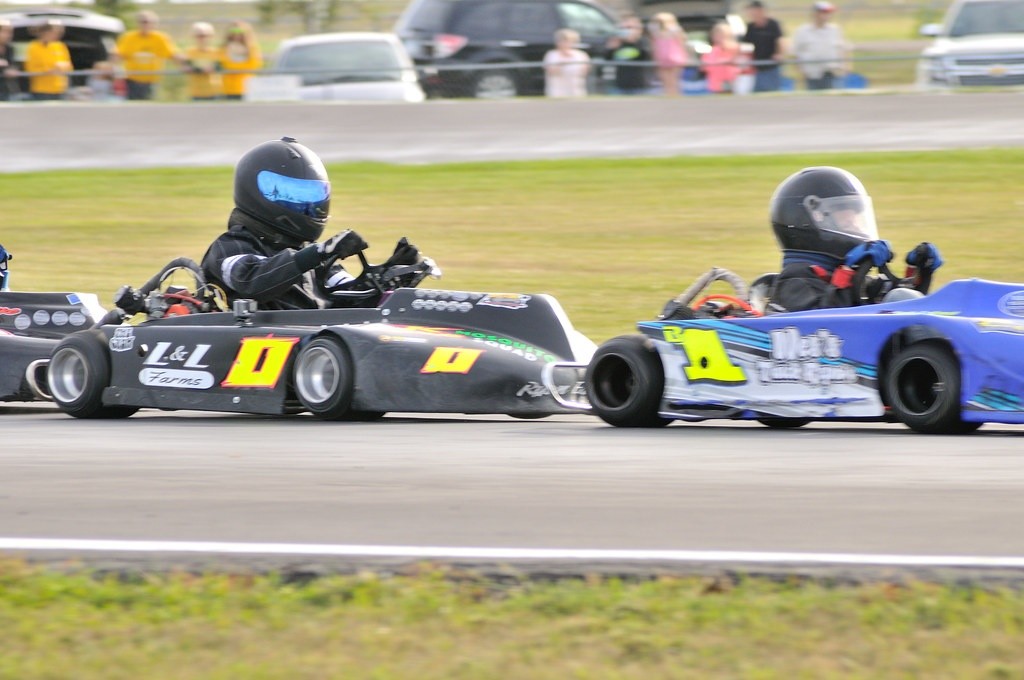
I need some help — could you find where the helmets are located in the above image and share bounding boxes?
[771,166,879,261]
[232,135,330,240]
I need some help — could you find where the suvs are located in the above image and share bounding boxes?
[910,0,1024,86]
[396,0,648,99]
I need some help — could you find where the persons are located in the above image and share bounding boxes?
[744,0,788,92]
[544,28,590,98]
[791,2,841,90]
[0,22,15,102]
[764,167,943,314]
[25,24,72,100]
[114,11,263,100]
[700,21,752,93]
[601,13,687,95]
[201,137,433,312]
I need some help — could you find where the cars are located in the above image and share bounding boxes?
[630,0,731,47]
[276,26,429,105]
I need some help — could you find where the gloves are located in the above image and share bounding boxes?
[905,242,942,272]
[844,240,892,269]
[381,237,420,290]
[315,227,369,265]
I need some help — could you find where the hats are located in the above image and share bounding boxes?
[193,22,215,37]
[813,2,835,13]
